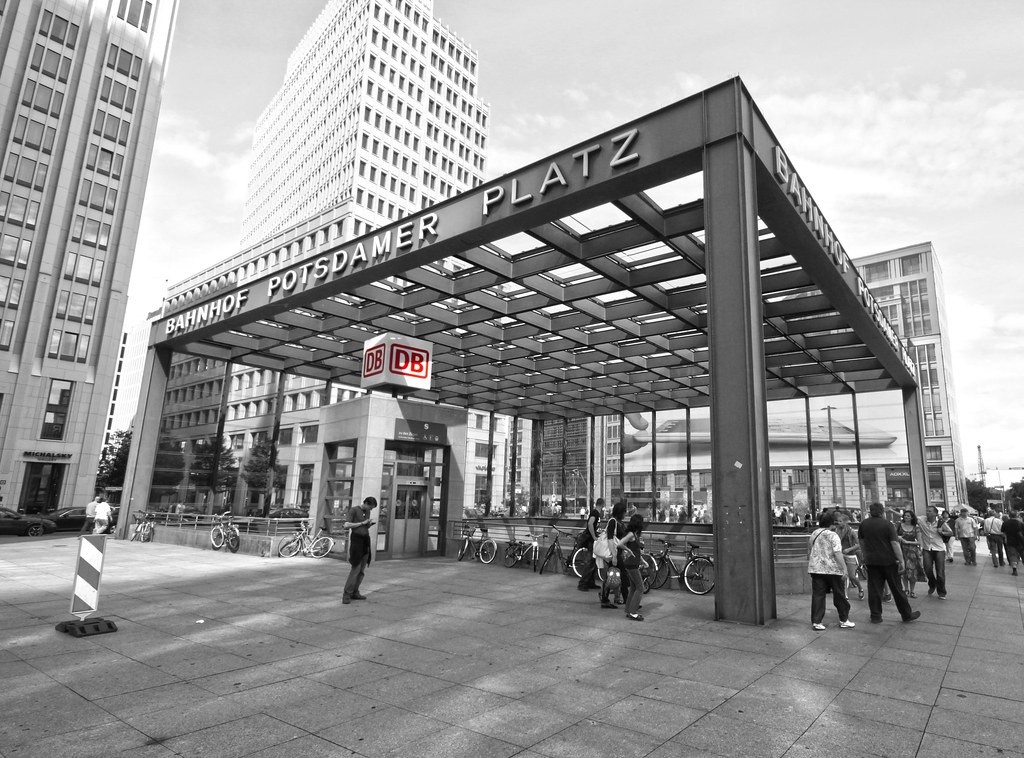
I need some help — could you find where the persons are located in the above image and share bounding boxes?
[896,509,924,599]
[772,504,906,526]
[807,513,856,630]
[886,507,953,600]
[836,515,865,601]
[858,503,922,624]
[882,579,892,601]
[580,503,712,521]
[940,505,1024,575]
[167,500,186,515]
[578,497,606,592]
[342,496,377,604]
[599,502,646,622]
[77,496,113,533]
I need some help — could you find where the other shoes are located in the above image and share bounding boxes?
[614,599,623,604]
[904,611,920,621]
[1000,561,1006,566]
[601,604,617,608]
[844,585,946,603]
[994,566,998,567]
[626,612,644,620]
[589,584,601,589]
[580,588,589,591]
[965,562,971,565]
[871,617,883,623]
[972,562,976,565]
[1012,568,1018,576]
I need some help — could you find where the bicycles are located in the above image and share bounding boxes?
[458,521,498,564]
[850,560,868,588]
[278,520,335,558]
[642,539,715,596]
[131,509,156,543]
[504,533,548,572]
[210,511,241,549]
[539,523,659,594]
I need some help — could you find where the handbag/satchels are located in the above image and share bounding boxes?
[577,533,591,549]
[937,518,950,542]
[593,518,617,563]
[987,533,991,539]
[596,556,606,568]
[605,567,621,589]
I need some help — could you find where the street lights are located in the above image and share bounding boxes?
[820,405,837,504]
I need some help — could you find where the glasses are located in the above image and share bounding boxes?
[624,510,626,513]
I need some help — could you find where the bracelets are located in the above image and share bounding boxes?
[361,521,364,526]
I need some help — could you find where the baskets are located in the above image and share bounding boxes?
[463,525,476,536]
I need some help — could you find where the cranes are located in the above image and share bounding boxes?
[986,466,1024,481]
[976,444,988,487]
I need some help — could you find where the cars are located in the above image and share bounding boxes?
[0,505,57,536]
[269,508,309,518]
[109,507,120,525]
[40,506,115,534]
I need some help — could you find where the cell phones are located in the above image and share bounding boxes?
[370,522,376,524]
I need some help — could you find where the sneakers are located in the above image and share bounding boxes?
[352,592,367,600]
[840,619,855,628]
[812,623,825,629]
[343,594,350,604]
